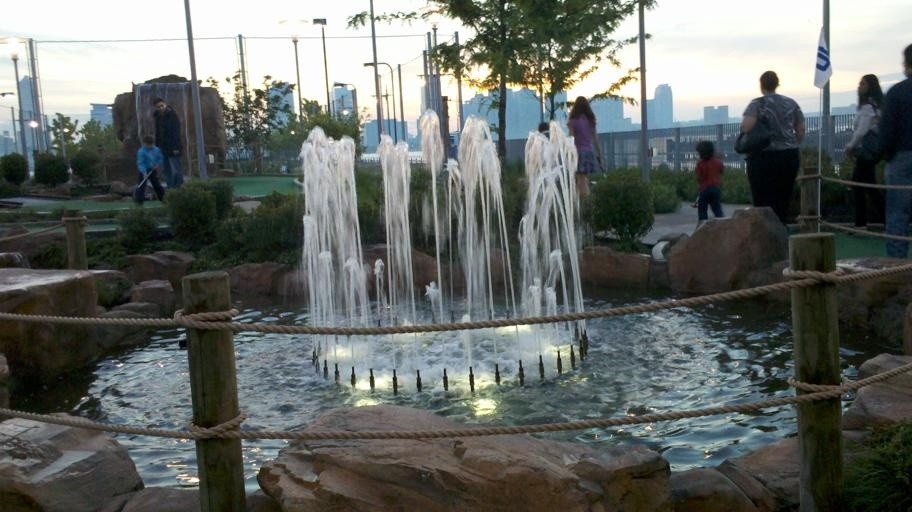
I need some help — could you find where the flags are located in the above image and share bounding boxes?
[814,28,832,89]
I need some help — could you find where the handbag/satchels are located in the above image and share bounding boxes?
[853,103,883,163]
[734,97,771,154]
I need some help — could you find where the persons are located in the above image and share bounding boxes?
[693,139,723,227]
[566,96,606,200]
[149,97,185,188]
[132,136,165,204]
[539,120,551,142]
[843,74,886,232]
[741,70,805,228]
[877,43,911,263]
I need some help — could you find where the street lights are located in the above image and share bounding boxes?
[334,81,361,149]
[364,62,397,146]
[291,34,306,139]
[313,17,333,120]
[55,112,66,157]
[12,118,38,156]
[10,50,30,181]
[431,20,442,139]
